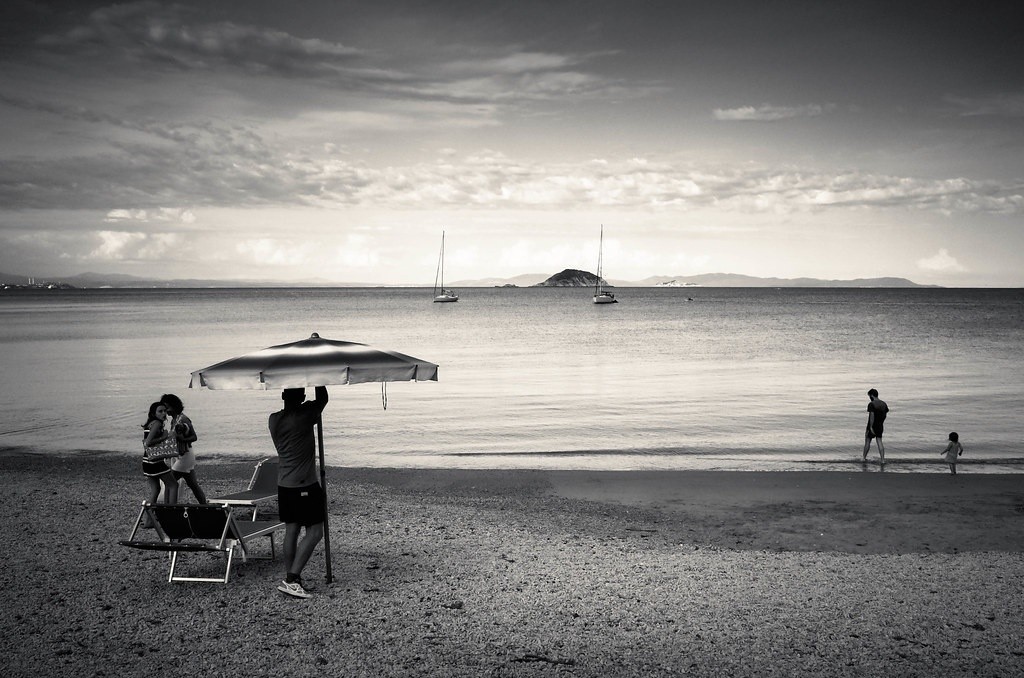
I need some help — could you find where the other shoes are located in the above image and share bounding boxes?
[140,518,161,529]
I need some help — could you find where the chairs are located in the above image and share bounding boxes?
[205,458,280,522]
[119,499,286,585]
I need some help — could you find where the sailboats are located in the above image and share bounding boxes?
[429,231,460,303]
[593,224,616,304]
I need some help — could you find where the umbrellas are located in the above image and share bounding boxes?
[188,332,439,587]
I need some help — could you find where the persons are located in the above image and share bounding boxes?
[141,401,178,527]
[941,432,963,474]
[860,389,889,464]
[269,387,328,599]
[161,394,206,504]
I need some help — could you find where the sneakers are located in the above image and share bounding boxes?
[278,580,312,598]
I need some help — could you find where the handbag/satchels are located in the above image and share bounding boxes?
[142,420,179,461]
